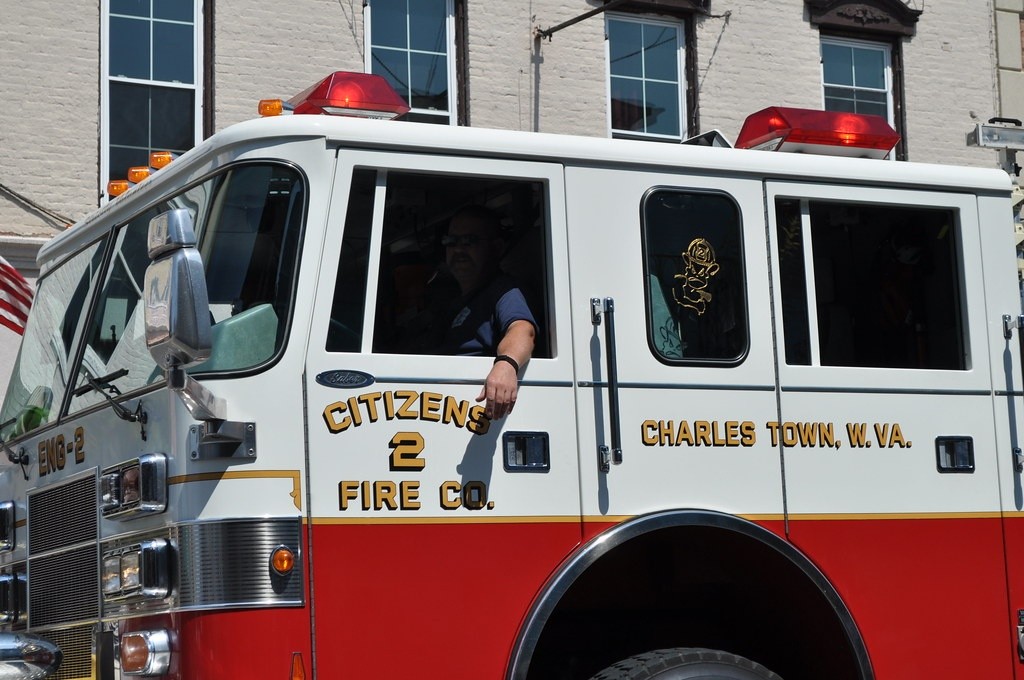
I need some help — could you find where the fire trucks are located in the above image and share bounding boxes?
[0,70,1024,679]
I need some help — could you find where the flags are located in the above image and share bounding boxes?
[0,255,35,336]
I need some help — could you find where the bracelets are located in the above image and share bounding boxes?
[494,355,519,374]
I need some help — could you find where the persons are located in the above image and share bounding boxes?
[399,205,539,419]
